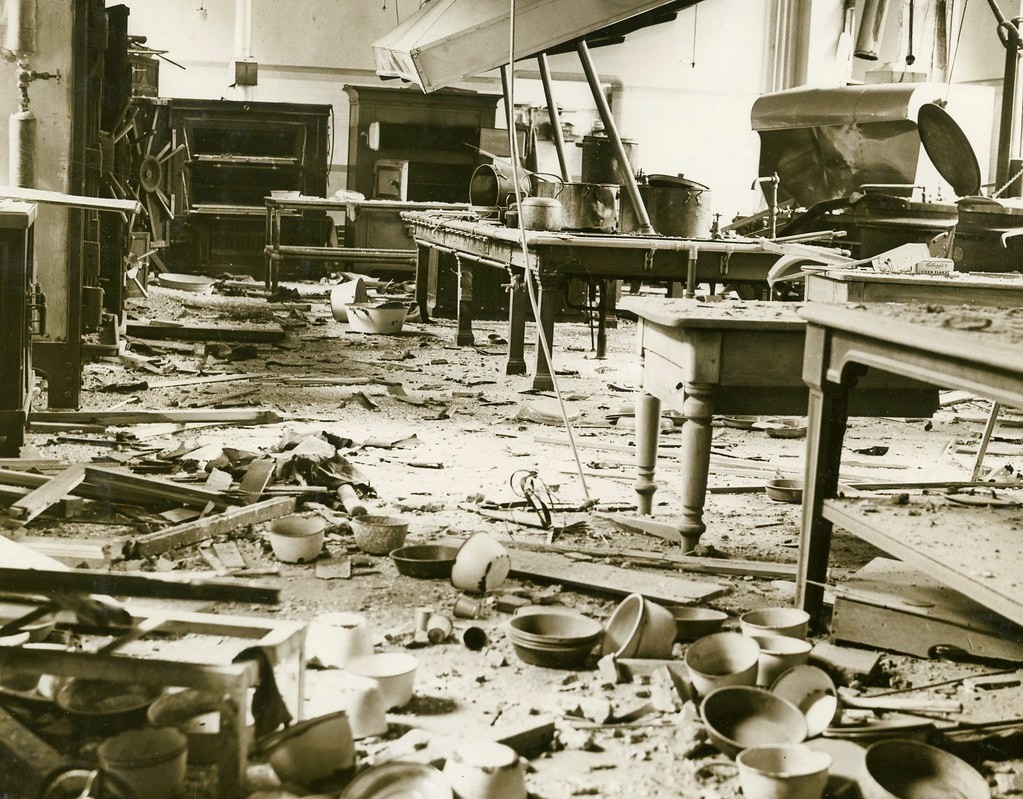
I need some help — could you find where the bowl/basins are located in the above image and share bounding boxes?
[54,671,257,799]
[259,612,525,799]
[349,515,409,555]
[267,514,328,570]
[505,591,989,799]
[158,273,216,297]
[389,531,510,595]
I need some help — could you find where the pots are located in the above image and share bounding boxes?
[330,278,409,334]
[469,164,712,240]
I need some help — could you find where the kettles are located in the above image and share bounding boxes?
[496,173,564,231]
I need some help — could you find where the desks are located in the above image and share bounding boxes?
[620,296,938,551]
[404,211,803,389]
[266,197,470,293]
[787,300,1023,633]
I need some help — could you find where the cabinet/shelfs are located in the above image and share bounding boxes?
[144,99,335,276]
[342,81,503,279]
